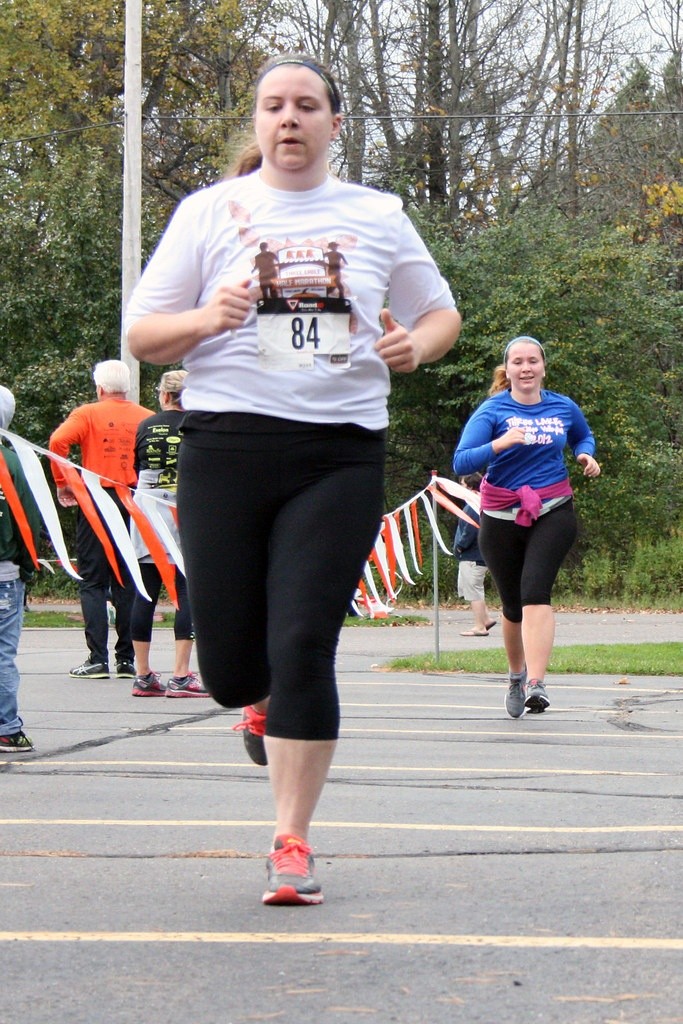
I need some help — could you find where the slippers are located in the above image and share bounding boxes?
[461,629,489,636]
[486,621,496,629]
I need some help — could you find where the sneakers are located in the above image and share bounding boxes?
[232,705,267,766]
[0,732,35,752]
[131,672,167,696]
[69,659,110,678]
[523,679,550,714]
[165,673,210,698]
[504,664,526,718]
[262,834,324,905]
[114,659,136,678]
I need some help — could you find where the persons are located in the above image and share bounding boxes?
[125,55,461,905]
[48,359,158,680]
[454,472,497,637]
[0,385,40,752]
[130,369,209,698]
[452,335,601,717]
